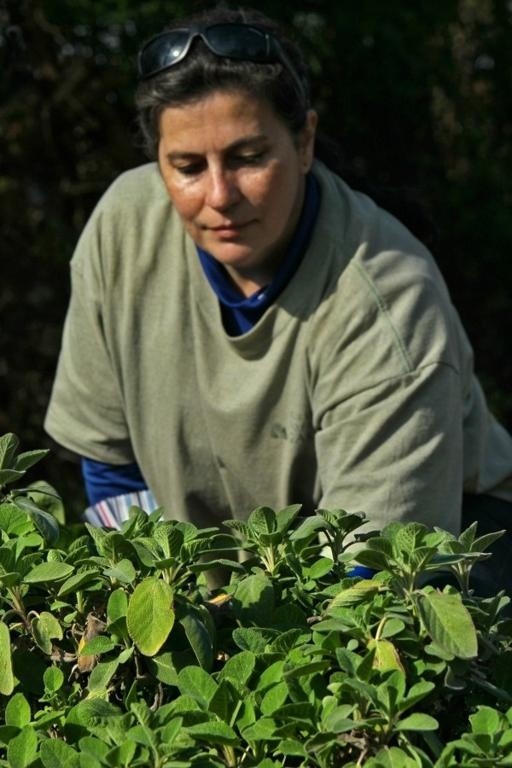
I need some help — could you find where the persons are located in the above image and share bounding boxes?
[45,2,512,603]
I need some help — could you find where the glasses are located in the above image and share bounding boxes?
[135,21,305,107]
